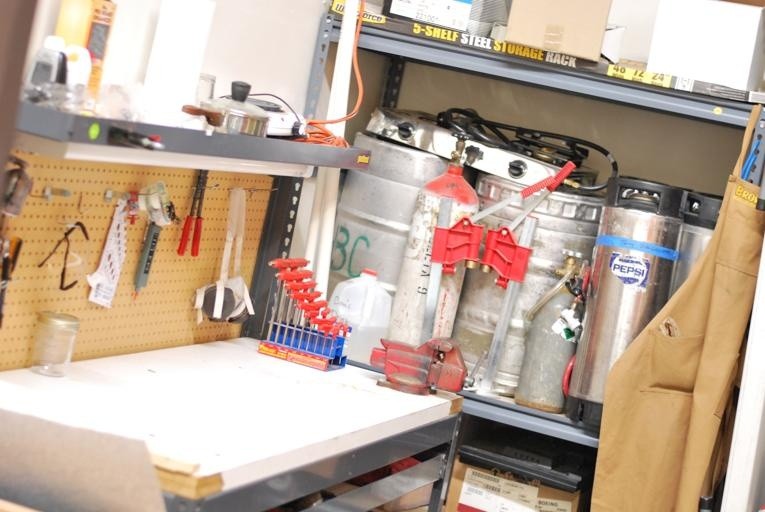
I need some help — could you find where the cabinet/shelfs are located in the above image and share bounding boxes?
[249,0,765,512]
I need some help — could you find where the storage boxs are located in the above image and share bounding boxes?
[645,0,763,92]
[506,0,614,62]
[444,453,589,512]
[388,0,508,41]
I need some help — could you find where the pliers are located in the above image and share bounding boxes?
[177,170,209,256]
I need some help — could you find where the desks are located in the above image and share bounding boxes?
[1,333,465,510]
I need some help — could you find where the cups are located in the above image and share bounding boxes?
[32,312,80,379]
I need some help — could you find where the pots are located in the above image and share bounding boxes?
[204,81,269,137]
